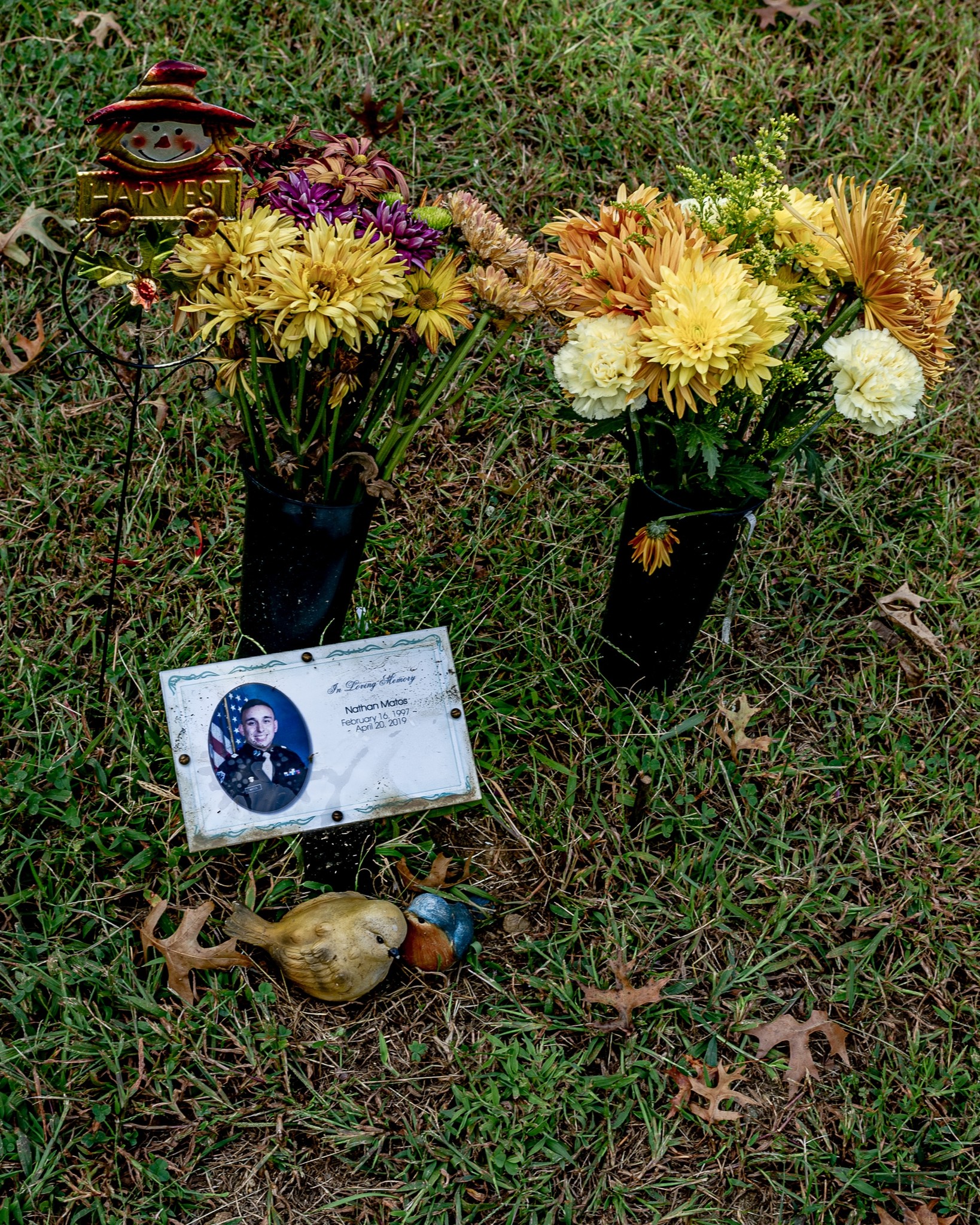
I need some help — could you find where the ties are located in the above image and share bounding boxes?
[261,752,273,782]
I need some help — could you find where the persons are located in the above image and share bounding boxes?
[213,700,306,813]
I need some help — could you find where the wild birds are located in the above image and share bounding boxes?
[223,889,470,1004]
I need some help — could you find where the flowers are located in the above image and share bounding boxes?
[541,113,960,576]
[81,129,555,481]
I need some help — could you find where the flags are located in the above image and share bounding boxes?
[208,689,248,767]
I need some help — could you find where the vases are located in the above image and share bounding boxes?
[238,458,376,658]
[598,473,751,699]
